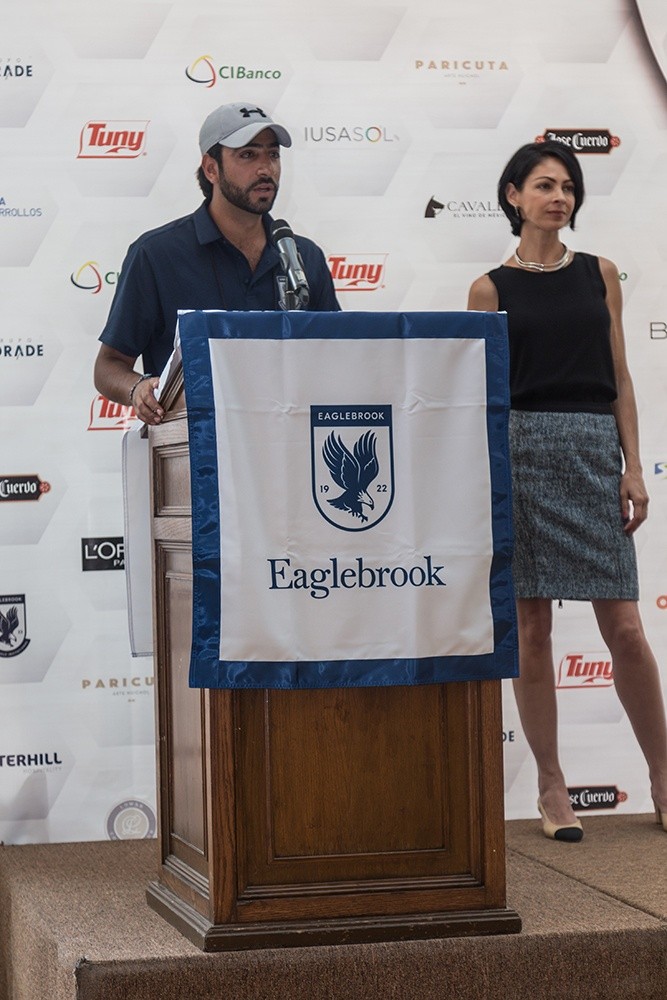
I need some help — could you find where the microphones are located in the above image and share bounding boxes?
[269,219,310,306]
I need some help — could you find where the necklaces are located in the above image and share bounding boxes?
[514,243,571,272]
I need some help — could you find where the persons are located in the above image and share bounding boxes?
[93,103,341,428]
[467,141,667,840]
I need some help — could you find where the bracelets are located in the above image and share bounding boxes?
[129,373,151,402]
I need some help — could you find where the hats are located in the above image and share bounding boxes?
[199,101,292,157]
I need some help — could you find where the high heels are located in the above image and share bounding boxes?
[538,796,584,843]
[651,787,667,832]
